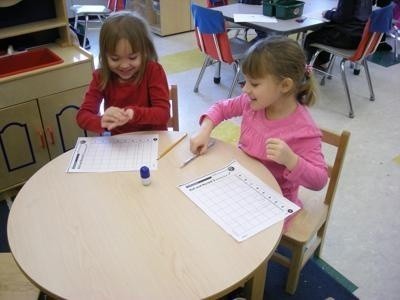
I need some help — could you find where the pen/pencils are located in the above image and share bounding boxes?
[180,142,215,167]
[157,131,187,160]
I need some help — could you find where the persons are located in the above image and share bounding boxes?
[304,1,369,72]
[76,12,169,135]
[193,35,328,232]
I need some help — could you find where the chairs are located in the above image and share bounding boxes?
[268,123,351,294]
[191,1,400,119]
[68,1,127,49]
[1,251,44,299]
[168,85,181,132]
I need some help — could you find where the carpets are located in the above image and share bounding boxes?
[1,187,360,300]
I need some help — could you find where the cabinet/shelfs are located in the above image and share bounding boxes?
[1,60,101,202]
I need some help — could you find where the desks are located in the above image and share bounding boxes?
[7,128,289,299]
